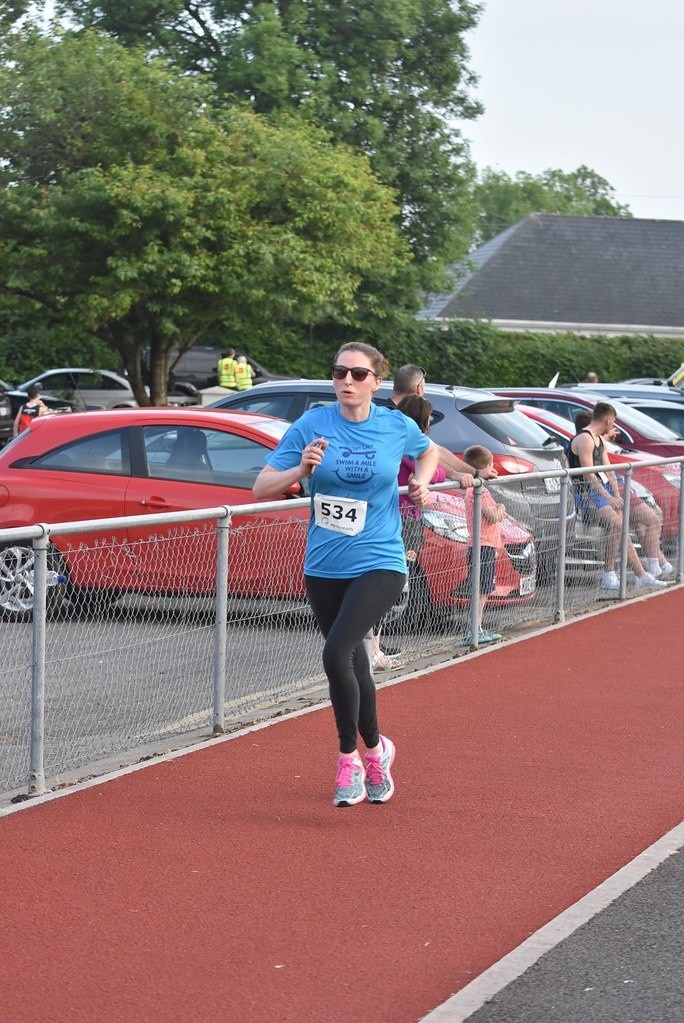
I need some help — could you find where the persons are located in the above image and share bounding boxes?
[253,342,439,806]
[218,348,255,411]
[13,388,49,439]
[370,363,678,674]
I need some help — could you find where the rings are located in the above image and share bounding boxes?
[424,498,427,502]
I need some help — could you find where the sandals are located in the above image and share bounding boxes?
[371,651,406,673]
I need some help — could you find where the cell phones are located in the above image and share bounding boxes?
[310,436,324,476]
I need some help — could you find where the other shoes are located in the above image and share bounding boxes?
[655,568,683,583]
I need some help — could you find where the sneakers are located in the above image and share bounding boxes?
[379,643,402,658]
[601,570,620,589]
[635,572,667,587]
[333,751,366,806]
[462,629,502,645]
[363,735,395,803]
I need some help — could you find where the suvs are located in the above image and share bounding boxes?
[139,343,301,398]
[105,378,578,589]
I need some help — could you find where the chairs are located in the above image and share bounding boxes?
[164,432,207,470]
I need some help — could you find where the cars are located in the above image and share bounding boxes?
[0,406,539,636]
[0,368,192,423]
[0,368,684,582]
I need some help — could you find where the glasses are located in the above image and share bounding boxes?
[332,365,377,381]
[417,367,427,389]
